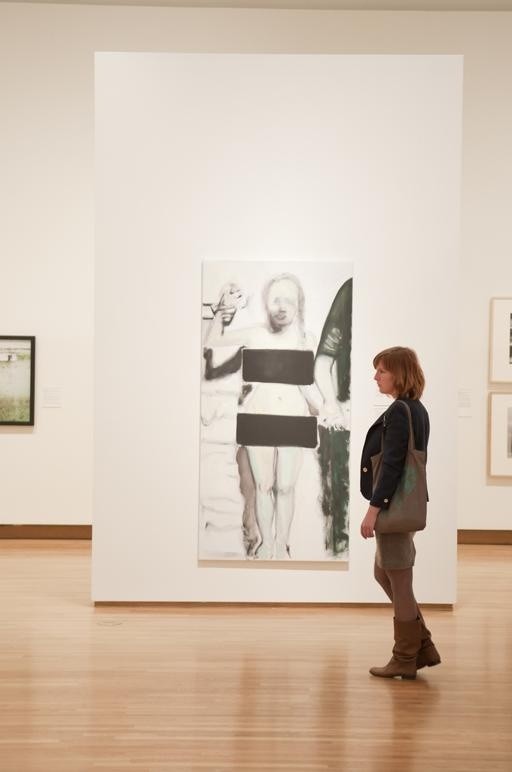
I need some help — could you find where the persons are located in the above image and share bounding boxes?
[313,278,352,558]
[205,275,332,561]
[361,346,440,680]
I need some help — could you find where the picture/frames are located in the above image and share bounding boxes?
[485,296,512,481]
[0,334,37,426]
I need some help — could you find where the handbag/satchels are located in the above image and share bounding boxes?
[370,450,426,533]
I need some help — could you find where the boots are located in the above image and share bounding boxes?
[369,615,441,681]
